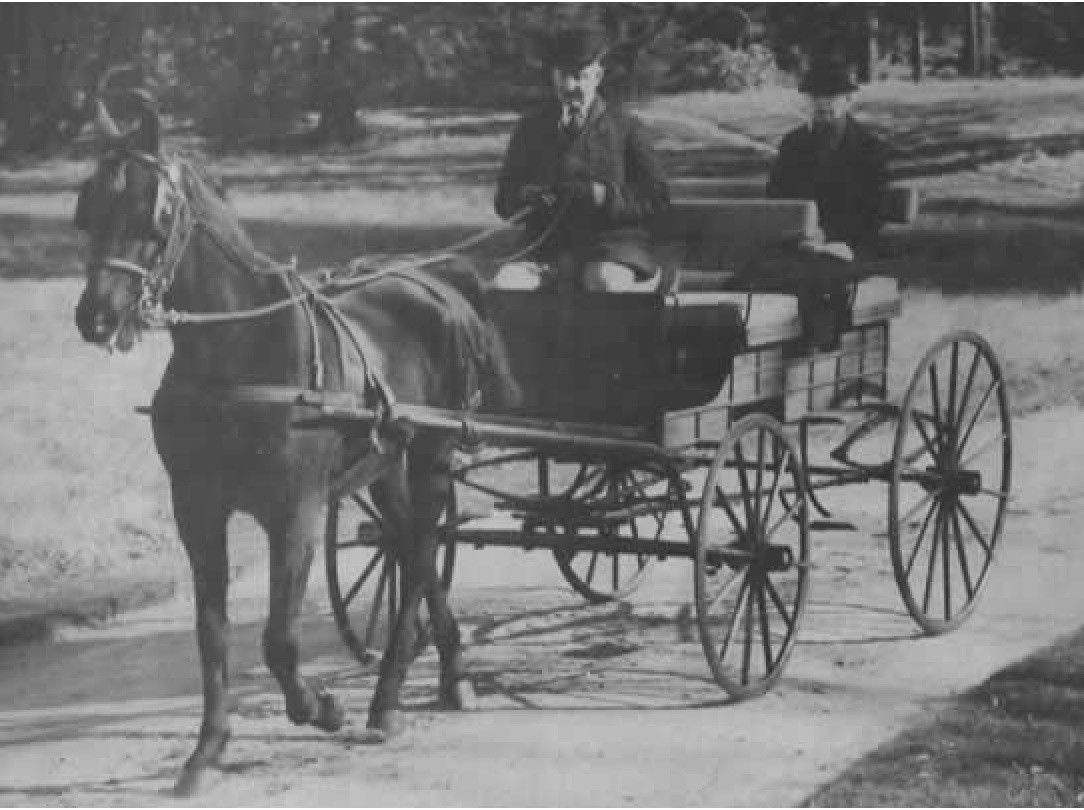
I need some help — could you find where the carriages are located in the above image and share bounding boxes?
[61,80,1016,795]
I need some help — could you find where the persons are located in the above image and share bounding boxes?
[493,23,673,291]
[765,60,890,248]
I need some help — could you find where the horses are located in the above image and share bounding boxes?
[72,85,519,797]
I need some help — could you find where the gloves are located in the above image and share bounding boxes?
[549,177,603,213]
[519,182,551,213]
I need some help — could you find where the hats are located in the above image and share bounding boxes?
[544,24,607,71]
[794,56,862,98]
